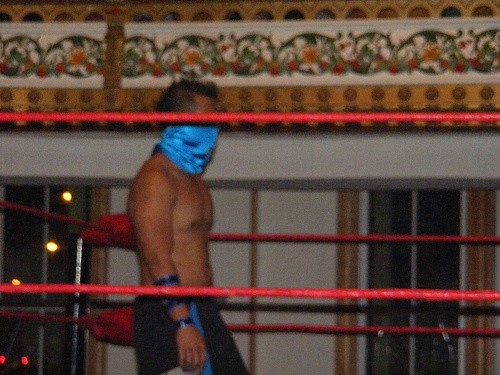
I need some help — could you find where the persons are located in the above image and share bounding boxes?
[126,78,250,375]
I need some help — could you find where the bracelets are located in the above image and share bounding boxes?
[174,317,194,330]
